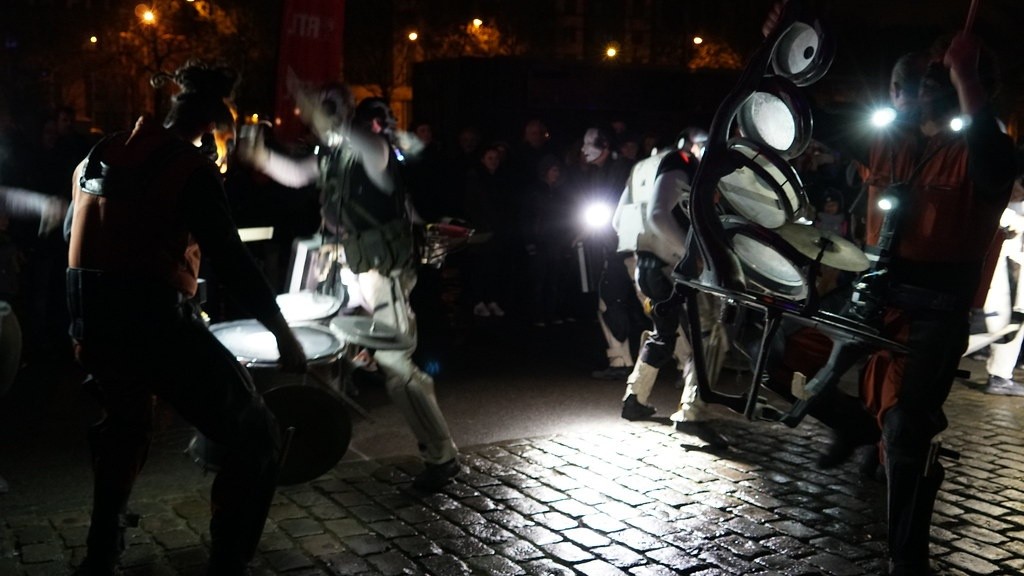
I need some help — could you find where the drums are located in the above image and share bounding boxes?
[715,19,823,304]
[272,291,340,322]
[186,320,349,486]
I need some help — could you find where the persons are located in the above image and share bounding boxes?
[235,65,461,495]
[0,92,307,576]
[614,129,861,448]
[400,109,661,382]
[846,30,1024,576]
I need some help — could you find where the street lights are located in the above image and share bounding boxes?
[142,10,163,101]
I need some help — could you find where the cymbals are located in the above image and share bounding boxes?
[775,221,871,273]
[328,315,415,351]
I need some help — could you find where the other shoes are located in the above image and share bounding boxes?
[813,421,883,468]
[622,394,657,421]
[590,367,633,382]
[427,461,463,479]
[984,375,1024,396]
[676,421,729,449]
[473,302,505,318]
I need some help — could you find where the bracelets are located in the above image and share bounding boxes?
[960,93,985,116]
